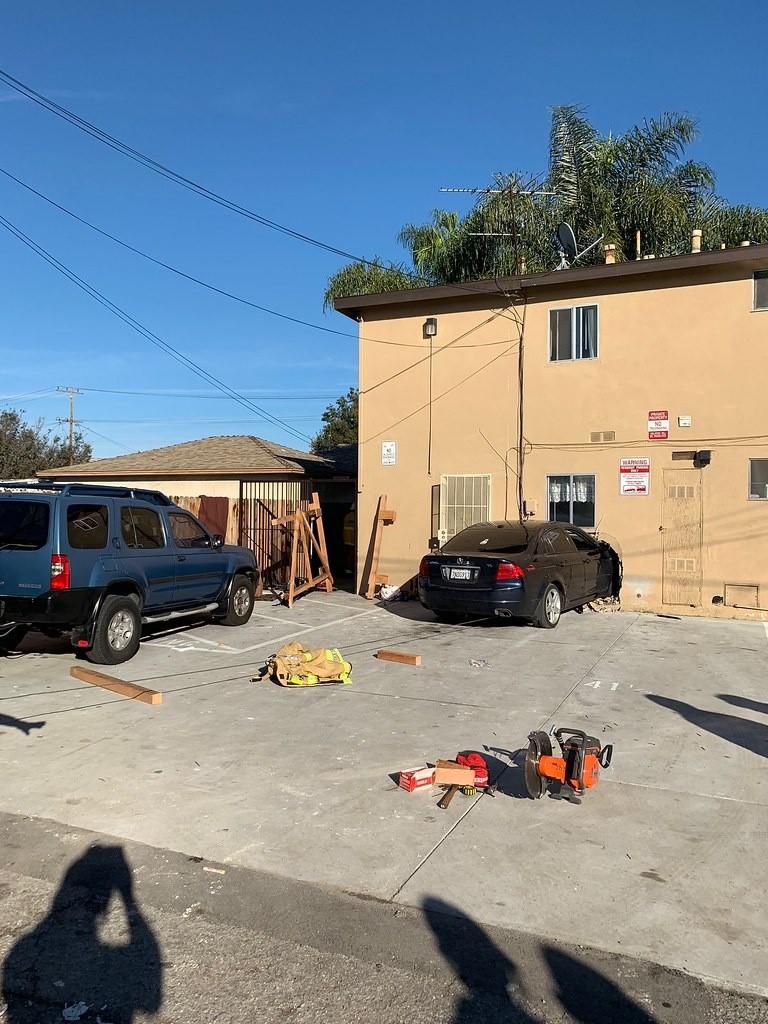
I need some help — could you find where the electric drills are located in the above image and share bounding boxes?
[525,724,613,805]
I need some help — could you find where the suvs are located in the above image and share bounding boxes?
[0,480,259,665]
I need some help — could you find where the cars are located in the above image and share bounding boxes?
[416,519,620,629]
